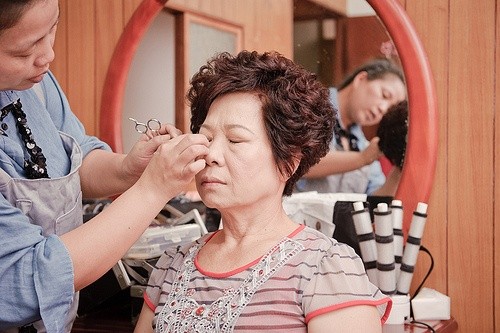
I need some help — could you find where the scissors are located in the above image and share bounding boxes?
[129,117,161,139]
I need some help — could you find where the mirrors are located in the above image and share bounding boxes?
[98,0,438,300]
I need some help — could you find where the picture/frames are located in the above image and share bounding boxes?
[173,10,247,190]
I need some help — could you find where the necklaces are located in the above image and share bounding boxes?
[332,118,359,152]
[11,97,51,179]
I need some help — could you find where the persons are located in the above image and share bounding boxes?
[0,0,211,333]
[378,98,410,169]
[131,51,393,333]
[289,55,407,195]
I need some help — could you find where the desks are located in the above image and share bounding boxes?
[70,306,458,333]
